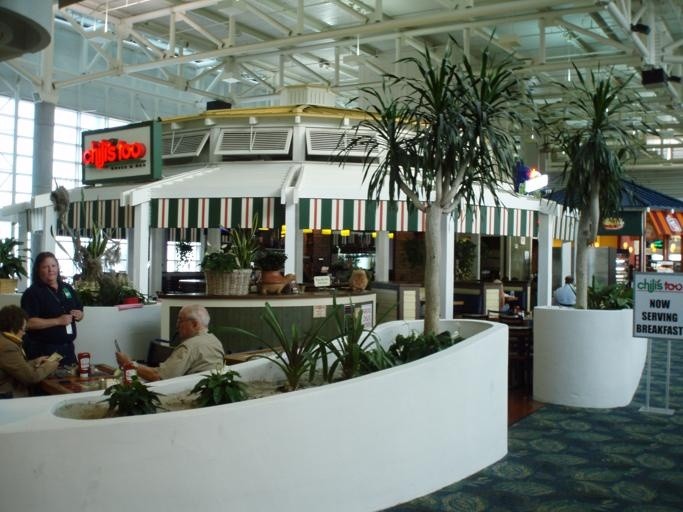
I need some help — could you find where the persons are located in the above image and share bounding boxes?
[552,276,576,307]
[486,266,518,313]
[20,250,84,365]
[0,303,60,401]
[114,304,227,383]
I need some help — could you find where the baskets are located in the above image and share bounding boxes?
[204,268,252,296]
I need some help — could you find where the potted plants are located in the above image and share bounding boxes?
[533,57,649,410]
